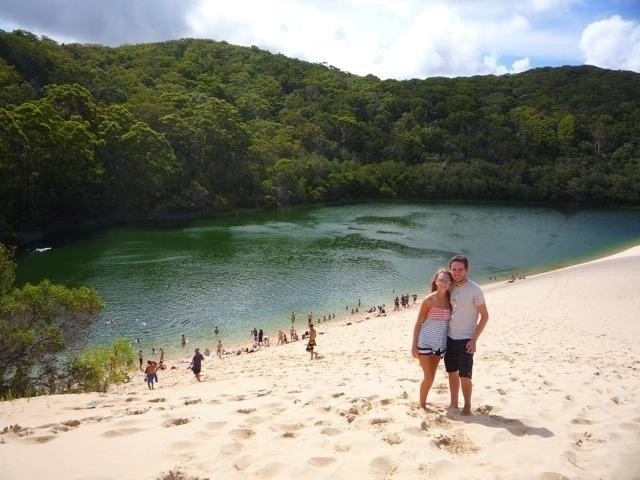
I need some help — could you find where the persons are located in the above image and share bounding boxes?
[441,255,489,417]
[181,334,186,347]
[412,269,455,411]
[247,289,419,359]
[216,340,223,358]
[189,348,204,382]
[137,347,165,390]
[214,326,220,332]
[203,348,210,356]
